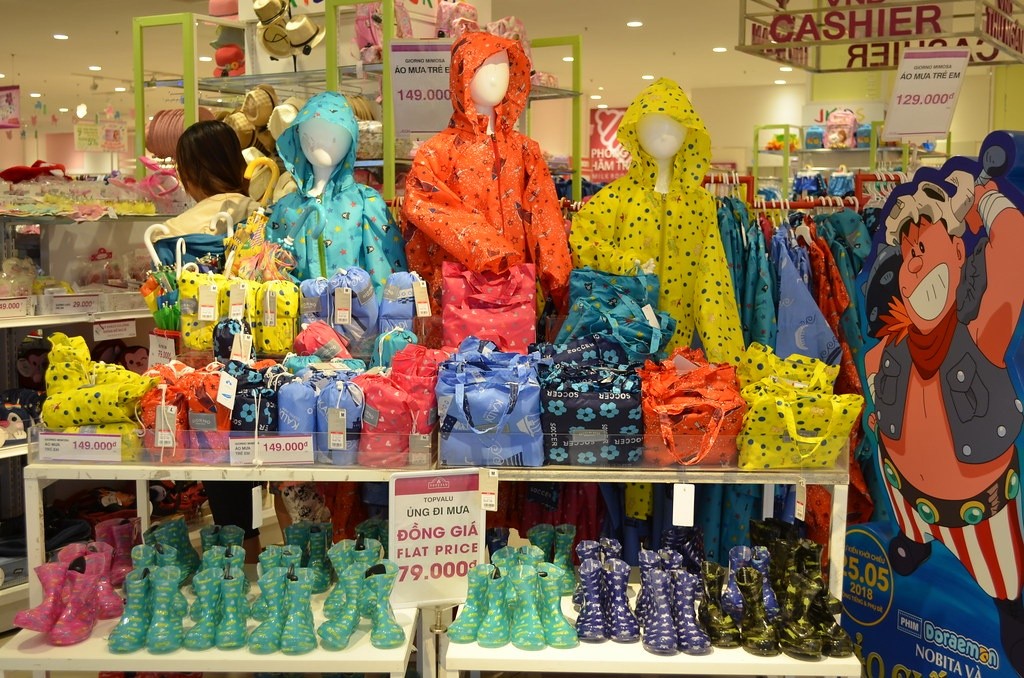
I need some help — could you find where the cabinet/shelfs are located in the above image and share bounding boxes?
[751,121,954,207]
[0,207,173,642]
[129,0,584,214]
[0,463,436,678]
[436,461,862,678]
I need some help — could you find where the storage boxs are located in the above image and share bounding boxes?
[100,287,144,311]
[0,296,34,318]
[33,292,100,314]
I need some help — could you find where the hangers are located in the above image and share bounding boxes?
[749,193,860,223]
[859,168,909,210]
[705,169,741,200]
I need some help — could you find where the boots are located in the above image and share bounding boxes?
[16,520,855,662]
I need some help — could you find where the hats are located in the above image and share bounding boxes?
[204,0,239,26]
[253,0,326,58]
[217,85,375,157]
[208,26,246,50]
[214,44,245,79]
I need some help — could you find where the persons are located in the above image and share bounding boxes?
[264,88,407,306]
[400,32,573,347]
[569,78,745,369]
[152,121,262,243]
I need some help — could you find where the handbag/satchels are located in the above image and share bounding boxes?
[40,261,864,469]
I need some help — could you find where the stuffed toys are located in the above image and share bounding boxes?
[0,337,149,448]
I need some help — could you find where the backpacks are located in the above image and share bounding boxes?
[434,2,482,41]
[805,108,878,148]
[351,1,415,62]
[530,71,558,89]
[485,16,533,73]
